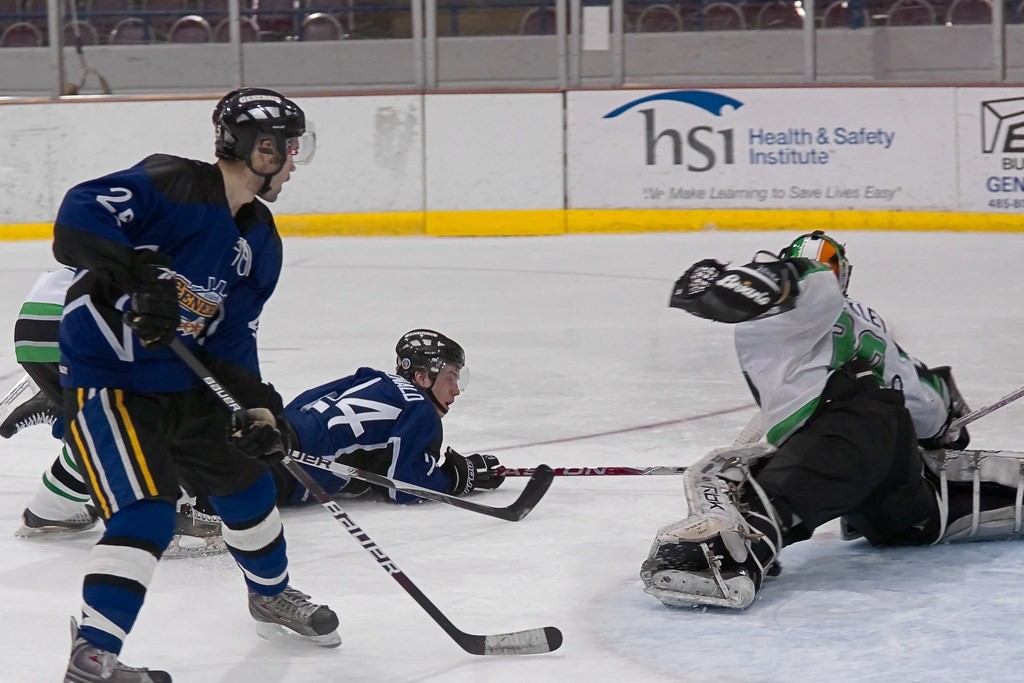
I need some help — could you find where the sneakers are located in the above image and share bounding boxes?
[162,498,229,563]
[652,569,756,610]
[0,373,65,440]
[14,503,99,538]
[63,617,172,683]
[247,586,341,648]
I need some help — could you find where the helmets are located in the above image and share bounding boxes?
[784,231,853,297]
[396,329,466,373]
[211,88,305,161]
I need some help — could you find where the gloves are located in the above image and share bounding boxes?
[122,269,180,351]
[479,453,504,490]
[225,406,278,453]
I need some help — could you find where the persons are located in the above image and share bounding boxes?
[0,328,507,506]
[50,87,341,681]
[13,265,229,561]
[640,229,1023,611]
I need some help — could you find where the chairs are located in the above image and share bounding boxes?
[63,20,99,46]
[198,0,248,29]
[109,17,157,45]
[0,0,22,32]
[251,0,301,38]
[213,15,262,43]
[518,6,556,35]
[168,14,213,43]
[885,0,937,26]
[26,0,66,44]
[141,0,189,40]
[0,22,43,48]
[83,0,135,43]
[703,2,746,31]
[755,0,802,29]
[303,0,355,33]
[946,0,992,24]
[302,12,345,40]
[820,0,870,28]
[636,4,683,32]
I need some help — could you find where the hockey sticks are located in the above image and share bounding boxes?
[498,409,766,477]
[121,309,565,658]
[949,385,1024,432]
[284,448,554,523]
